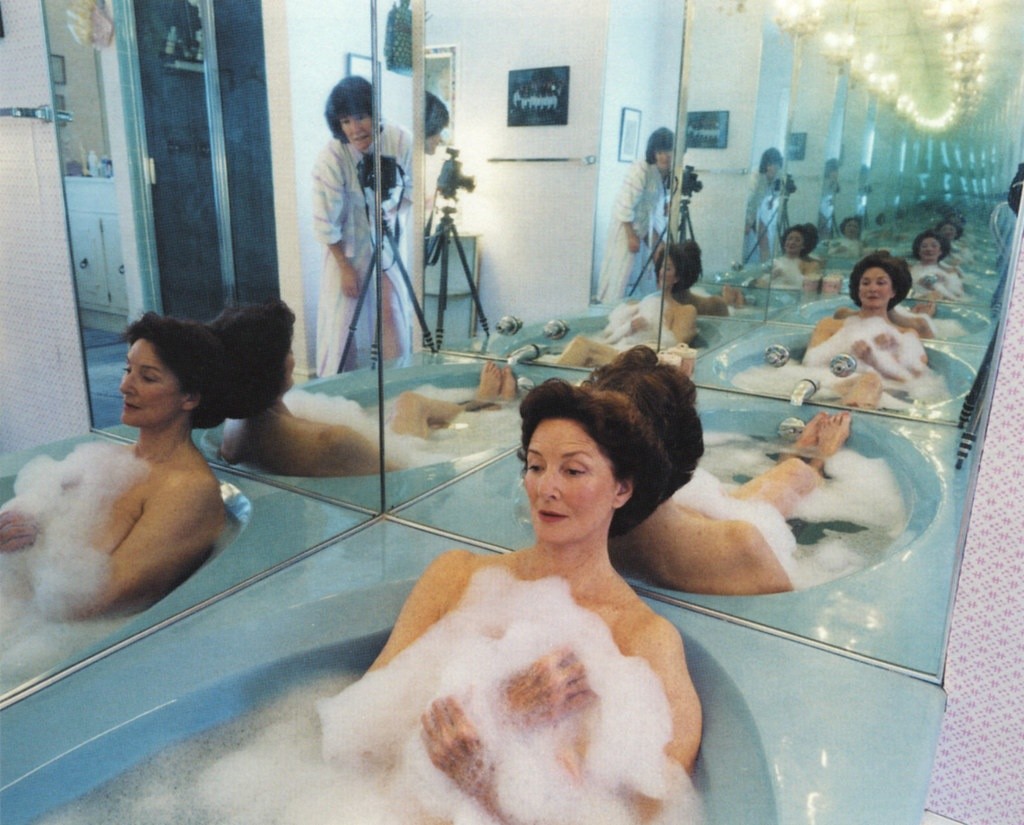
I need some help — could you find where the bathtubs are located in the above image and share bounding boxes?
[1,449,253,632]
[268,194,1012,598]
[1,612,780,823]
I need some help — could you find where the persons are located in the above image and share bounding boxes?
[209,301,515,477]
[0,314,222,617]
[362,378,702,823]
[311,77,411,378]
[558,129,967,594]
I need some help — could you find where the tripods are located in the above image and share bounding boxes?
[335,194,491,374]
[626,200,694,297]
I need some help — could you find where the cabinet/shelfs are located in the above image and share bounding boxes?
[61,176,128,318]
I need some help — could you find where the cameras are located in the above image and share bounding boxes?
[786,175,797,195]
[437,147,475,202]
[681,166,703,198]
[357,152,397,193]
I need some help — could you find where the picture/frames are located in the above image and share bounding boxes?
[347,51,371,82]
[618,105,643,165]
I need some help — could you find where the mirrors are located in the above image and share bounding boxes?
[0,0,384,711]
[42,0,1023,516]
[367,0,1023,692]
